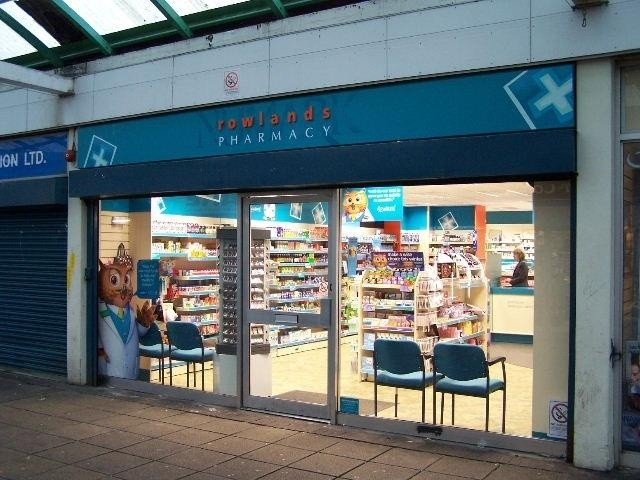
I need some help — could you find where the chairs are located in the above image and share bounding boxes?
[373,339,444,424]
[433,343,506,434]
[136,321,178,385]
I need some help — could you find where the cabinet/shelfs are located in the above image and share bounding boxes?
[217,235,330,349]
[487,234,533,276]
[270,239,314,300]
[152,231,216,341]
[342,233,491,380]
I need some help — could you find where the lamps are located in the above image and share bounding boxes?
[433,343,506,434]
[373,339,444,424]
[136,321,178,385]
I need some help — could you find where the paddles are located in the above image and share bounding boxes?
[270,390,399,416]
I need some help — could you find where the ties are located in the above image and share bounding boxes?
[484,253,502,288]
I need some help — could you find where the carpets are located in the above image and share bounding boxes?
[270,390,399,416]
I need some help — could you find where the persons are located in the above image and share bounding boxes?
[505,249,528,287]
